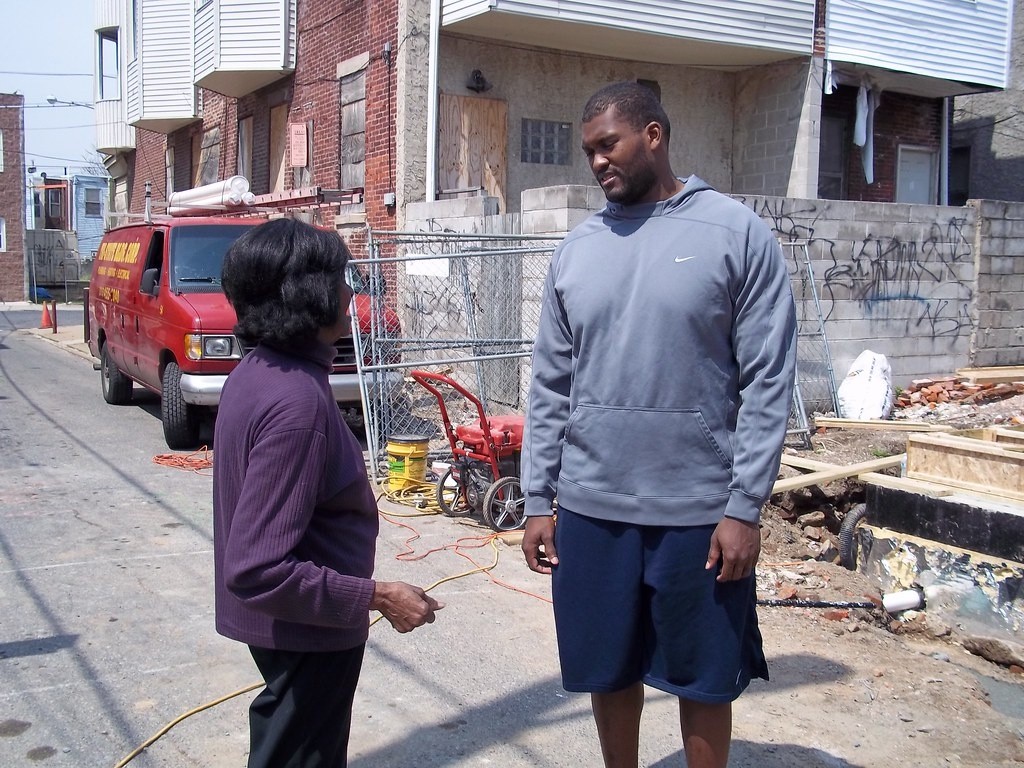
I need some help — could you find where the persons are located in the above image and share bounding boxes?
[212,218,445,768]
[520,82,798,768]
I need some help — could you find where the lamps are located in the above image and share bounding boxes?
[466,70,493,94]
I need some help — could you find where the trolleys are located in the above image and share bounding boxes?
[408,369,530,532]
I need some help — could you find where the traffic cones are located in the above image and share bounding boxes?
[38,301,54,329]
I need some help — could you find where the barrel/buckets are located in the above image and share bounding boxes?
[386,434,428,492]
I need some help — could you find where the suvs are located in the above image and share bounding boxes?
[87,180,404,454]
